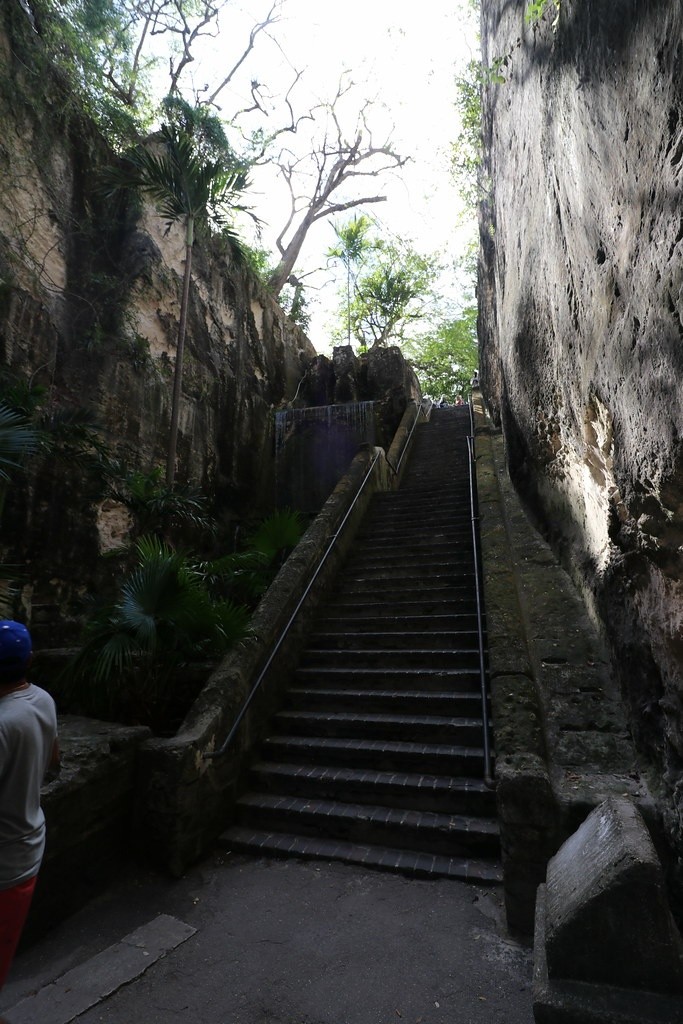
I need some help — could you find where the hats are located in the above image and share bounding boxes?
[0,620,31,670]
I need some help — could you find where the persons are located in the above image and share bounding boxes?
[1,619,63,987]
